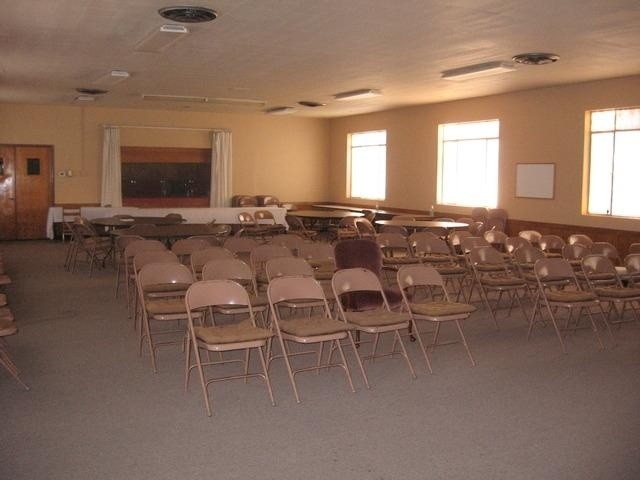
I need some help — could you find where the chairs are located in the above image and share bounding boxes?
[58,193,640,419]
[0,271,33,391]
